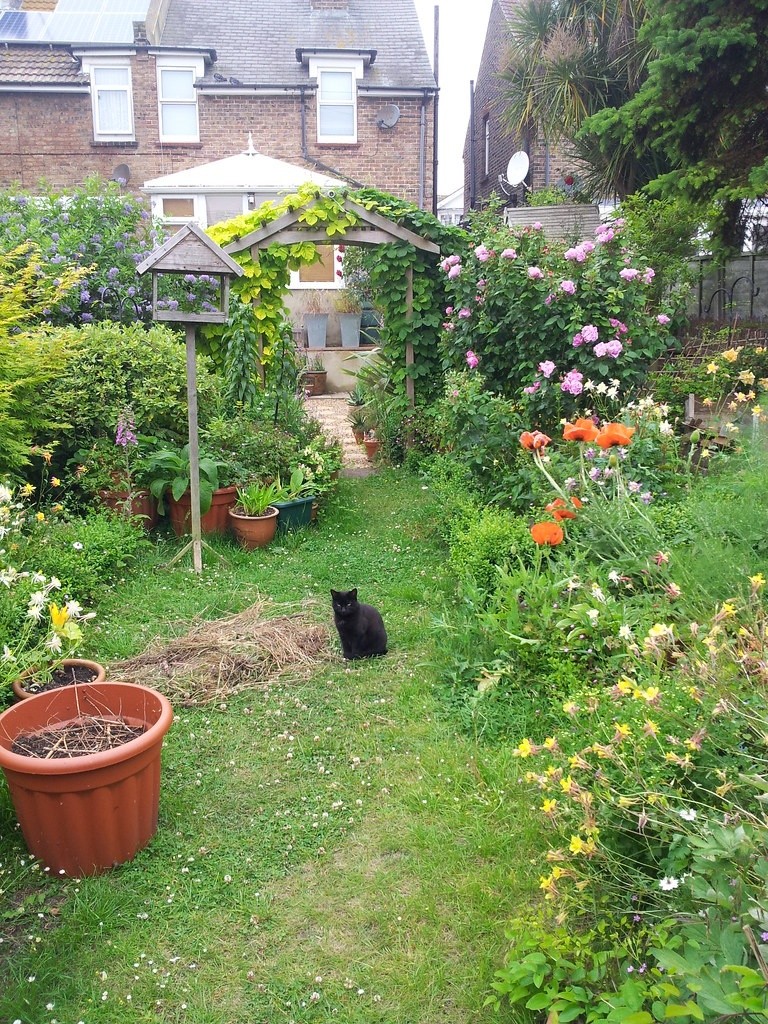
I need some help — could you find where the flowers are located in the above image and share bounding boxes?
[296,347,325,370]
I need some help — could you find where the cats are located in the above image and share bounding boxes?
[330,588,388,661]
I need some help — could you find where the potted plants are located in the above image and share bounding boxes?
[12,657,106,706]
[314,450,340,481]
[304,481,319,520]
[334,287,363,347]
[363,429,382,459]
[346,411,365,445]
[148,443,238,536]
[0,681,174,880]
[300,288,330,347]
[269,469,315,531]
[228,481,290,549]
[298,371,327,396]
[91,448,160,532]
